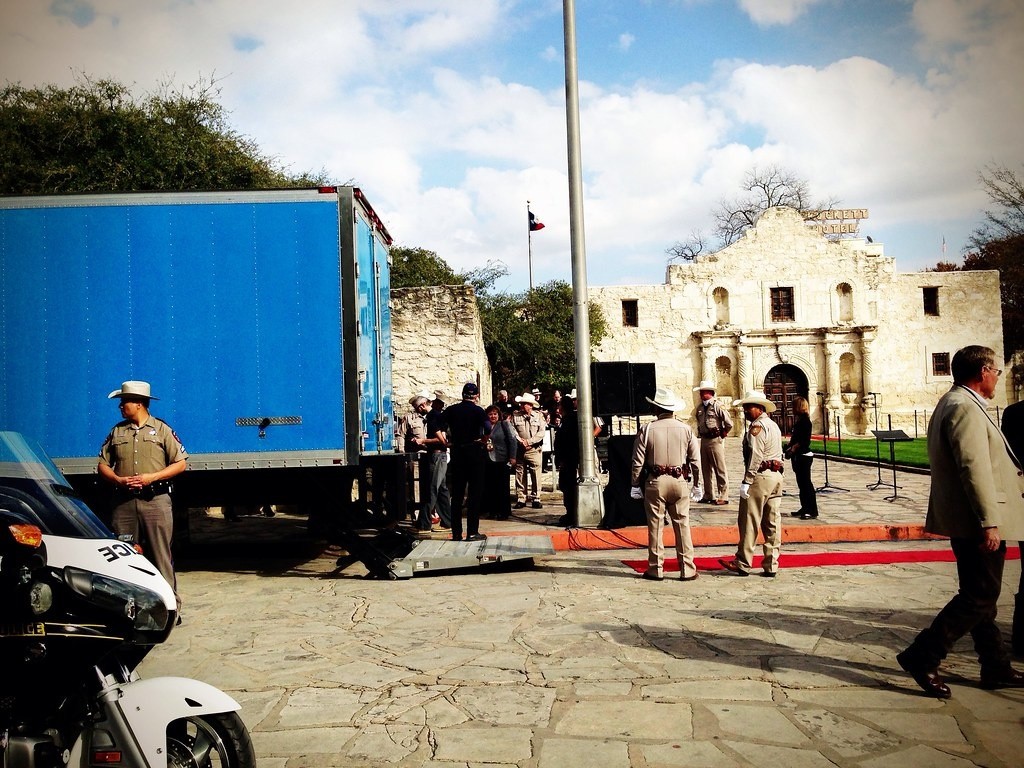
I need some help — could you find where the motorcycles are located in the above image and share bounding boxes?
[0,431,256,768]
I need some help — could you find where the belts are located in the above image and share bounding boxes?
[428,450,446,454]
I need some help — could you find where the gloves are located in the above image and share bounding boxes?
[631,486,645,499]
[690,486,704,502]
[741,482,750,499]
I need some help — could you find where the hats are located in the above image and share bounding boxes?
[733,390,776,413]
[531,389,542,395]
[434,390,451,403]
[693,380,718,391]
[107,381,161,400]
[409,391,437,407]
[565,390,577,399]
[645,389,686,411]
[515,392,540,409]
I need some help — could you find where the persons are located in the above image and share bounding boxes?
[566,389,605,471]
[513,393,546,509]
[480,404,517,521]
[718,390,784,577]
[97,381,189,626]
[432,383,492,542]
[554,396,581,526]
[630,388,703,581]
[392,388,452,533]
[999,352,1024,660]
[693,380,733,505]
[782,397,819,520]
[896,345,1024,697]
[497,388,564,429]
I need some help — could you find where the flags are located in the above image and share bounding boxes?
[528,210,545,231]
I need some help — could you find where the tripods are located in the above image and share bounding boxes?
[816,394,850,492]
[865,395,915,503]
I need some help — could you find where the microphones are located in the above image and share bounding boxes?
[817,392,829,395]
[868,392,881,395]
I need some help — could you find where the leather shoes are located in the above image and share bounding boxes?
[764,571,775,577]
[896,649,951,698]
[718,558,749,576]
[979,668,1024,689]
[466,533,486,542]
[452,534,463,541]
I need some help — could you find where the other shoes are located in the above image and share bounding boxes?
[431,514,440,526]
[408,526,431,534]
[514,502,525,509]
[533,501,543,510]
[432,525,452,532]
[801,511,817,520]
[698,497,712,503]
[643,571,664,581]
[713,500,728,504]
[791,508,804,516]
[681,575,698,581]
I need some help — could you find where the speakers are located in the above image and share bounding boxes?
[591,361,657,417]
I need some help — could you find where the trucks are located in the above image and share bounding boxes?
[0,185,393,535]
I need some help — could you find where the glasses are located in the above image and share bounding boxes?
[985,364,1002,376]
